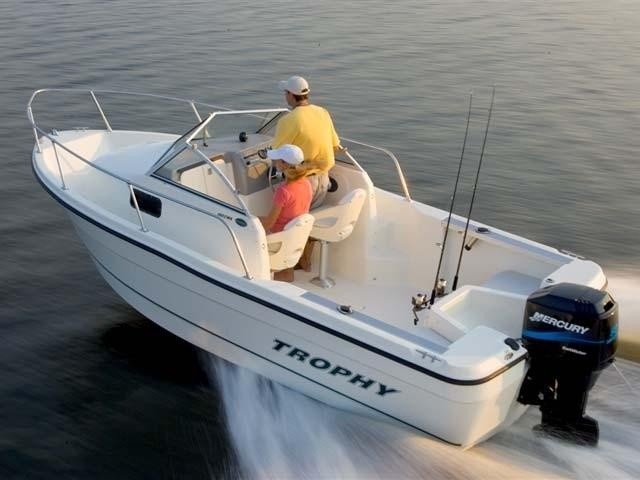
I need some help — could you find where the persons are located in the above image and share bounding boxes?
[258,76,341,283]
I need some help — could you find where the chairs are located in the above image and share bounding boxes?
[267,187,368,289]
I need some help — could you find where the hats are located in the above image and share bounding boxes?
[266,144,304,165]
[278,76,310,96]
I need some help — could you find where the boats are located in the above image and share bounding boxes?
[25,86,620,453]
